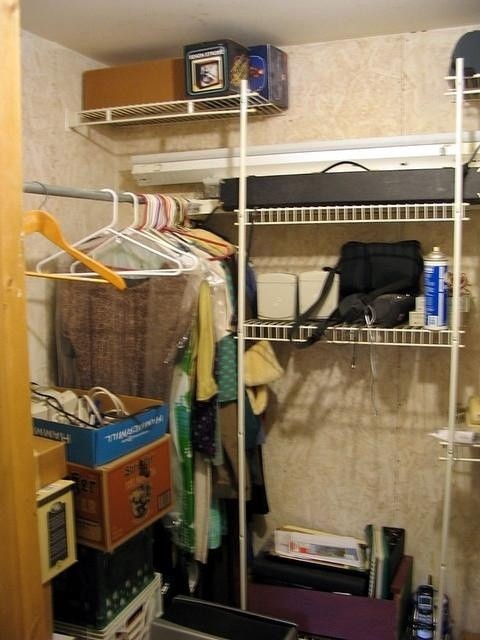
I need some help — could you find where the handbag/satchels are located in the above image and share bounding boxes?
[338,240,424,301]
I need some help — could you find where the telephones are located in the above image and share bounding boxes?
[410,576,450,640]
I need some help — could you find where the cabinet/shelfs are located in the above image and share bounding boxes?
[64,57,480,640]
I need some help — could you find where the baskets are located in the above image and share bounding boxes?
[53,570,165,639]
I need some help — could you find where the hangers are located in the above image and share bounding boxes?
[14,189,231,293]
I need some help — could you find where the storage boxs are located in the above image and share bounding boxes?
[29,384,170,470]
[183,40,249,110]
[32,436,67,491]
[248,43,288,108]
[80,59,199,110]
[64,434,175,551]
[236,554,415,640]
[30,478,83,589]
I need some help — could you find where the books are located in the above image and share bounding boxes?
[270,523,392,601]
